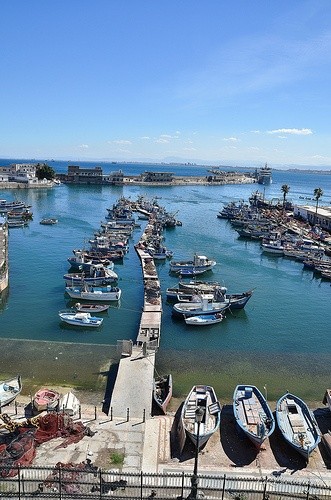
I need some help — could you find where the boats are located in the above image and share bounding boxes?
[312,388,331,454]
[170,291,231,316]
[58,392,81,415]
[217,162,331,280]
[71,303,110,313]
[34,387,60,411]
[0,427,39,480]
[152,373,173,413]
[179,279,228,294]
[0,197,59,294]
[65,285,121,300]
[233,385,277,446]
[169,253,216,273]
[166,287,256,310]
[63,194,190,287]
[0,375,23,408]
[276,389,323,457]
[175,269,206,277]
[182,313,227,326]
[58,312,103,328]
